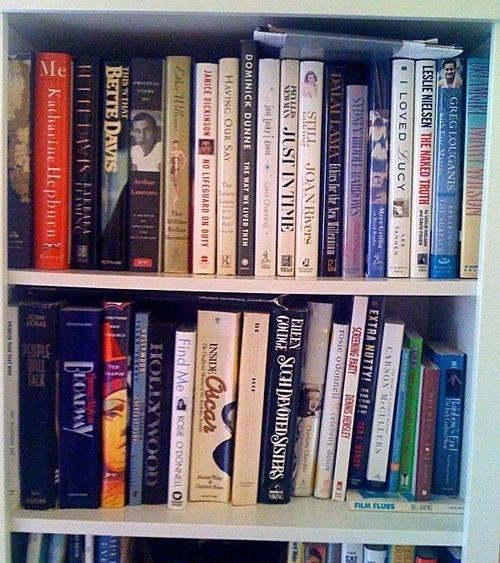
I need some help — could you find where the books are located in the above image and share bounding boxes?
[8,23,489,279]
[6,295,467,512]
[27,533,461,563]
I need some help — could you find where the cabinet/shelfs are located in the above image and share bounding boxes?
[0,0,500,563]
